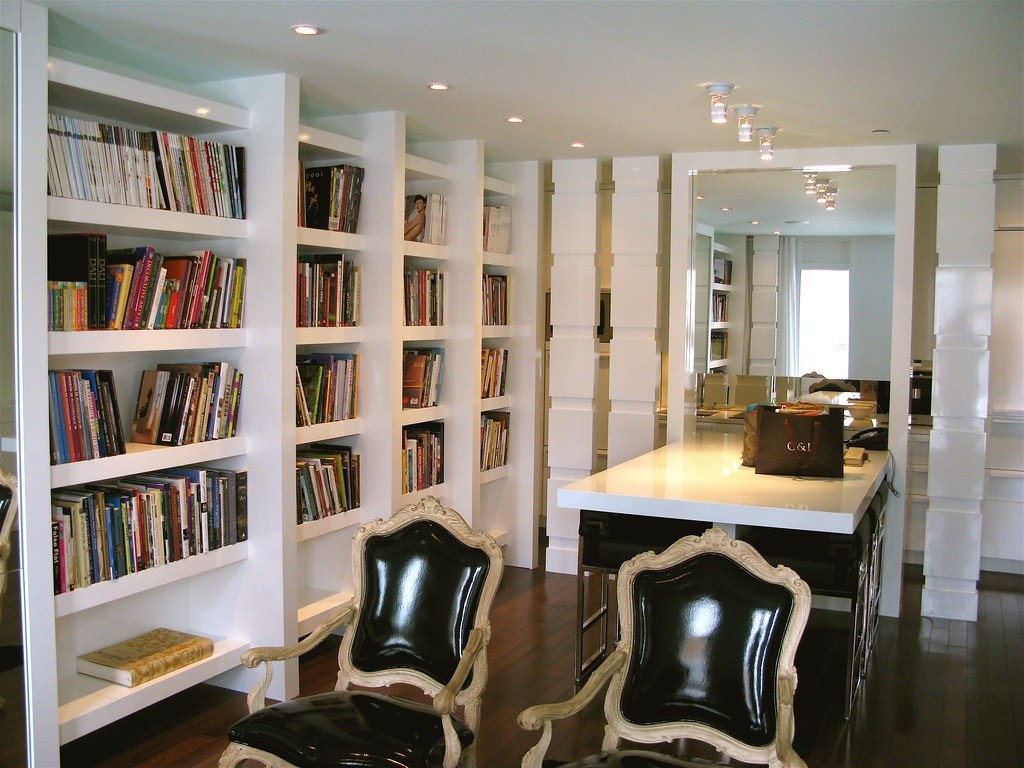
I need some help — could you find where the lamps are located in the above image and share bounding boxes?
[816,180,829,203]
[733,106,760,143]
[755,128,779,161]
[707,84,733,124]
[825,188,837,211]
[803,172,818,194]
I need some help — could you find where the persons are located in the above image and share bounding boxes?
[404,195,427,241]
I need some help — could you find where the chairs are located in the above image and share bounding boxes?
[516,525,813,768]
[217,496,504,768]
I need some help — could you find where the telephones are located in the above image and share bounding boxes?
[845,426,888,450]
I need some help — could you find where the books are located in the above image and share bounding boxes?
[482,274,507,325]
[403,347,445,408]
[76,628,214,688]
[713,259,733,285]
[46,112,247,219]
[47,233,247,331]
[297,159,365,233]
[481,347,508,398]
[296,443,360,525]
[480,411,510,472]
[296,352,360,427]
[402,422,444,495]
[404,193,445,245]
[296,253,361,328]
[129,361,244,446]
[483,206,512,254]
[844,447,865,467]
[404,266,445,326]
[51,465,248,595]
[709,370,727,374]
[710,332,728,361]
[712,295,730,322]
[48,368,127,466]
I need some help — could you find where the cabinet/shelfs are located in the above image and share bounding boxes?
[706,243,731,410]
[0,0,542,768]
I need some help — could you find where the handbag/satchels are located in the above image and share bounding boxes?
[756,402,843,480]
[741,411,756,467]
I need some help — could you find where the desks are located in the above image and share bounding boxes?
[556,431,892,725]
